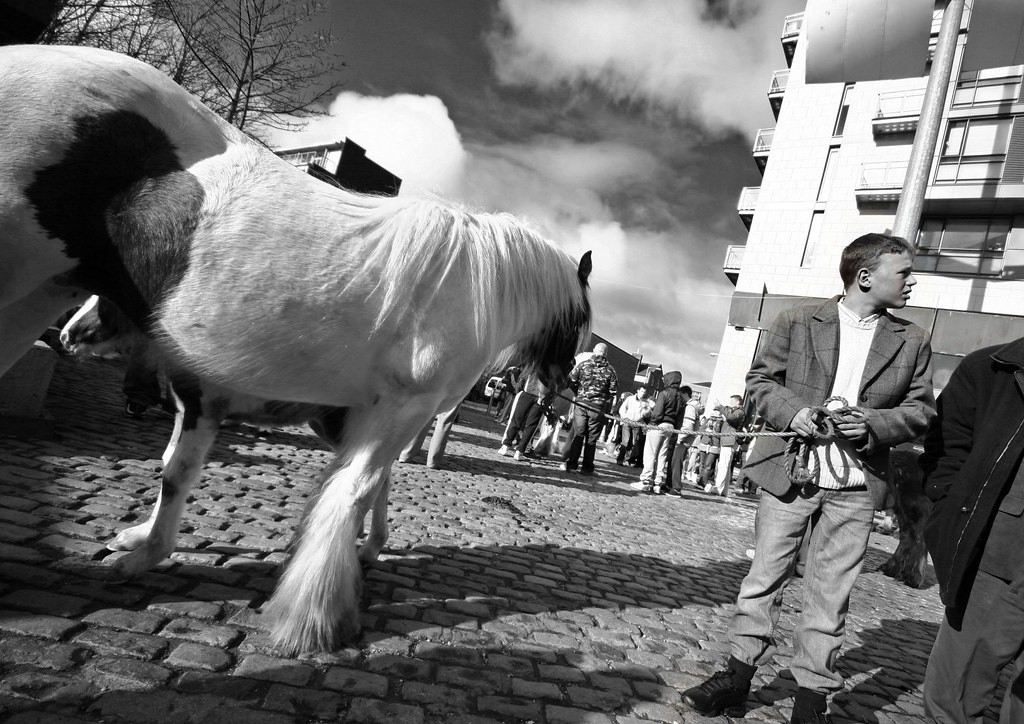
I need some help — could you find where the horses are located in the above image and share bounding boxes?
[0,44,593,660]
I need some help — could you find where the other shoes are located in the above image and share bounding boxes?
[630,480,650,491]
[162,405,176,420]
[618,459,622,466]
[628,462,636,467]
[665,489,682,498]
[567,467,581,473]
[498,446,515,456]
[514,452,528,461]
[124,400,148,420]
[581,469,599,477]
[652,485,661,494]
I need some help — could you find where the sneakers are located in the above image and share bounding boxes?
[791,703,831,724]
[681,670,751,718]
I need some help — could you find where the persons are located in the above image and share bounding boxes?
[495,343,762,496]
[681,232,935,724]
[122,368,176,419]
[397,396,462,469]
[916,339,1024,724]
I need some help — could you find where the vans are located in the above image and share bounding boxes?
[481,376,507,405]
